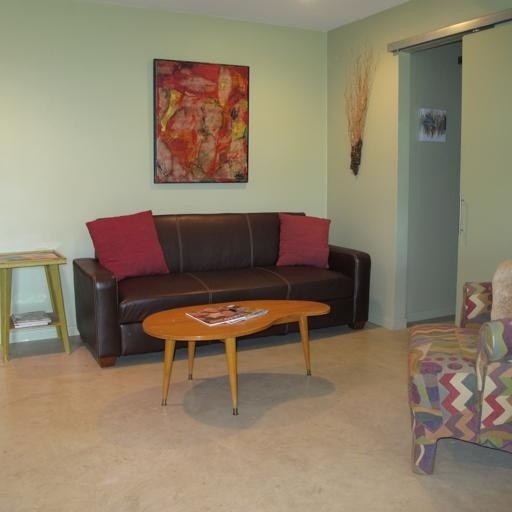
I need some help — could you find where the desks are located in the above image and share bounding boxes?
[0,248,72,362]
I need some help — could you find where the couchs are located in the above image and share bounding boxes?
[73,209,370,369]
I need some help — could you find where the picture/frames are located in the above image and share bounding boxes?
[152,59,250,184]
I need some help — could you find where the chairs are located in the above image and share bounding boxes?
[405,258,512,477]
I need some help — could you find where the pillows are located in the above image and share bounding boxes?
[489,258,512,322]
[84,209,172,282]
[275,211,332,271]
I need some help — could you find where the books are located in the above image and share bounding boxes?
[11,310,51,329]
[187,303,245,326]
[225,304,269,325]
